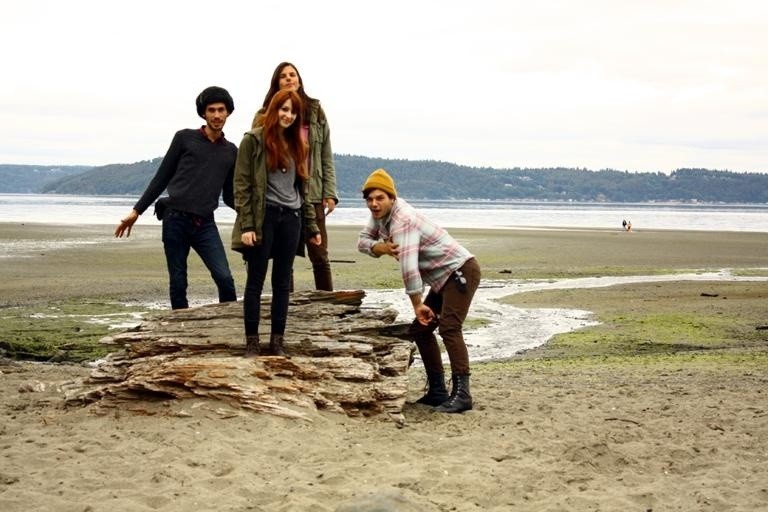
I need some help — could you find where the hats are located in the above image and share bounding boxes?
[361,167,397,201]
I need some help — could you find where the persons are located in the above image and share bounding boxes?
[231,88,323,358]
[251,61,339,292]
[115,86,241,309]
[627,220,632,232]
[622,219,627,229]
[357,169,481,413]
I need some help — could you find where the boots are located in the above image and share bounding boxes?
[415,374,448,406]
[244,334,261,358]
[429,371,472,414]
[268,333,291,359]
